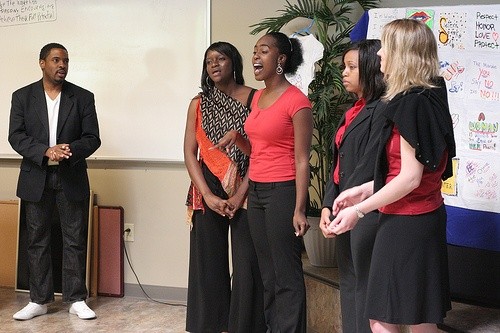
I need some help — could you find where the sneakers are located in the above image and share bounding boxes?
[68,299,96,319]
[12,301,47,320]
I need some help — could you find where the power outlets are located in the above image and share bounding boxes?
[124,223,134,241]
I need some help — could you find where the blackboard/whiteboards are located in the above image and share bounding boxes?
[1,1,212,164]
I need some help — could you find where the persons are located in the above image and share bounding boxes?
[319,38,389,333]
[8,43,102,320]
[327,18,457,333]
[209,32,313,333]
[184,42,267,333]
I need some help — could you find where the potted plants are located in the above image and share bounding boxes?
[249,0,381,267]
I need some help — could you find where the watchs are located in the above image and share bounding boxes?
[354,205,364,219]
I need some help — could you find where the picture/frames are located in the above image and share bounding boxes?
[14,190,124,298]
